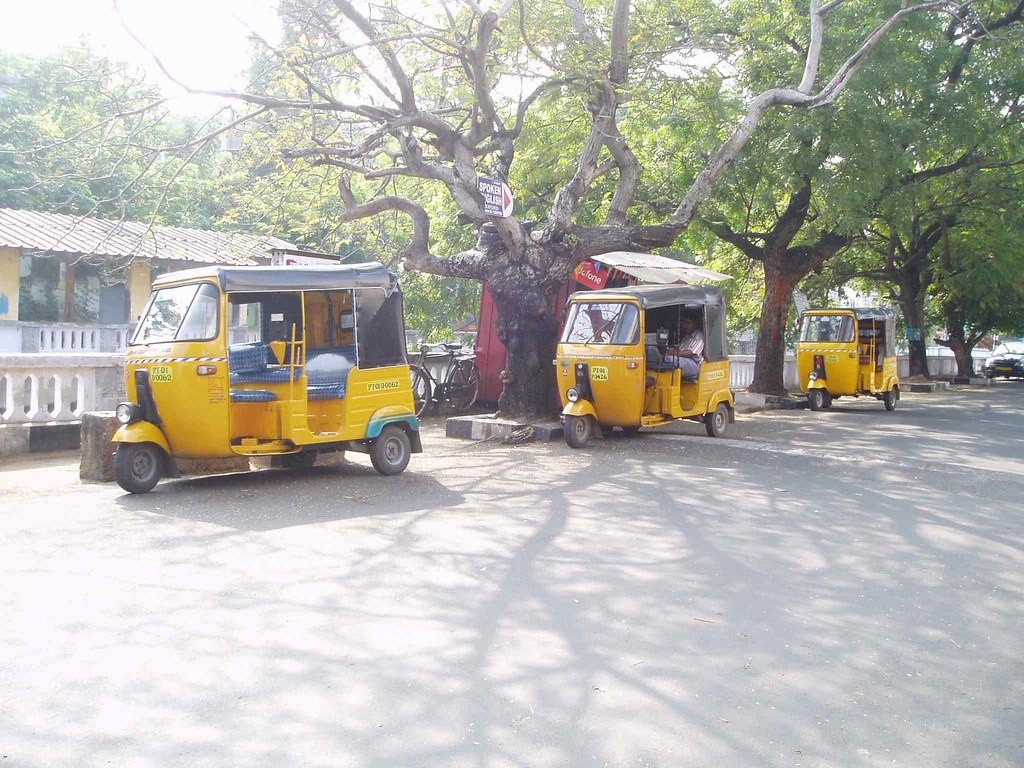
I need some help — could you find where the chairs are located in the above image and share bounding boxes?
[229,340,282,441]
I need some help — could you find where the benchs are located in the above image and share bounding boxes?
[288,344,359,432]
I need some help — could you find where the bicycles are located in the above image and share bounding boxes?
[406,340,481,417]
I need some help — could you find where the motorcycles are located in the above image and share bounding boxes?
[795,307,903,410]
[111,259,426,495]
[554,283,737,452]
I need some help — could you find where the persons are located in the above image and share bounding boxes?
[664,314,706,381]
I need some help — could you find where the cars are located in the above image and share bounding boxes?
[985,339,1024,379]
